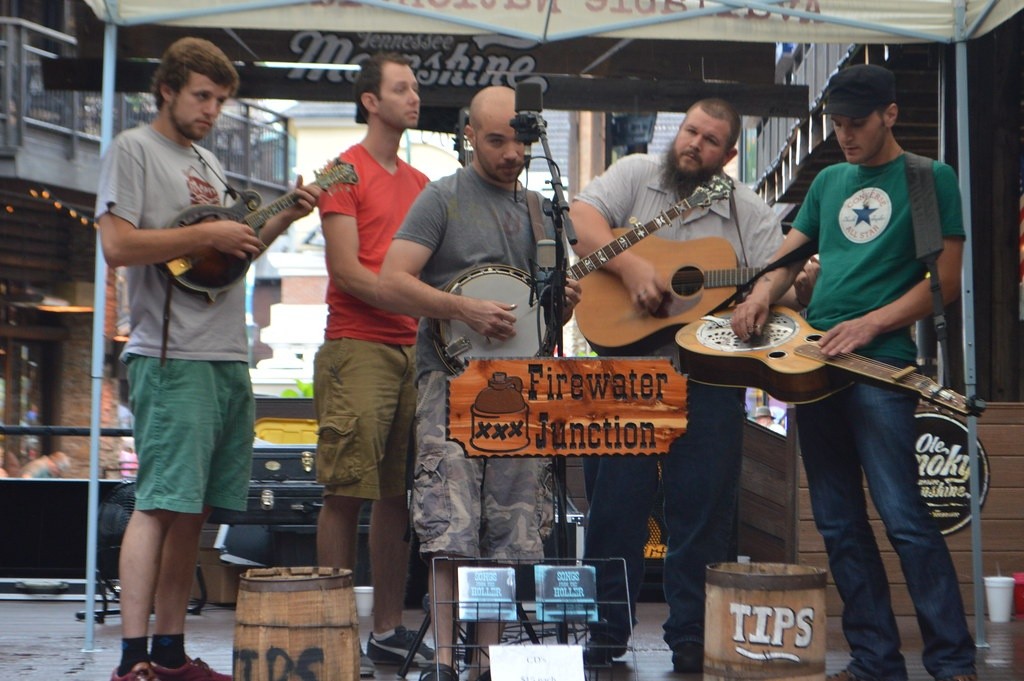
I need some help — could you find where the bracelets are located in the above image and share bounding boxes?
[796,296,809,309]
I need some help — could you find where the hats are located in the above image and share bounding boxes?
[821,64,897,117]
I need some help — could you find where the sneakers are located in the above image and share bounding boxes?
[671,642,704,673]
[826,670,860,681]
[583,634,627,670]
[149,657,232,681]
[367,624,435,668]
[110,656,161,681]
[359,638,376,676]
[952,673,977,681]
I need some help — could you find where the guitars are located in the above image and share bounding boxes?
[432,175,736,380]
[162,156,361,301]
[675,306,986,419]
[573,227,763,356]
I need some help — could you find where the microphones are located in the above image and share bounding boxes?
[513,82,543,170]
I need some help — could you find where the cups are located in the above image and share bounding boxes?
[984,576,1015,622]
[1012,572,1024,620]
[354,586,374,617]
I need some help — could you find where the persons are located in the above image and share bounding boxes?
[94,38,320,680]
[312,53,435,676]
[565,98,820,668]
[376,86,582,681]
[731,63,979,681]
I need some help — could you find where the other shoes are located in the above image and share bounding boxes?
[419,663,459,681]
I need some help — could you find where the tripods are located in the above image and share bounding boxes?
[397,596,540,677]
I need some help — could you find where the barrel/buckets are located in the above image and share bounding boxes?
[703,563,828,681]
[231,566,362,681]
